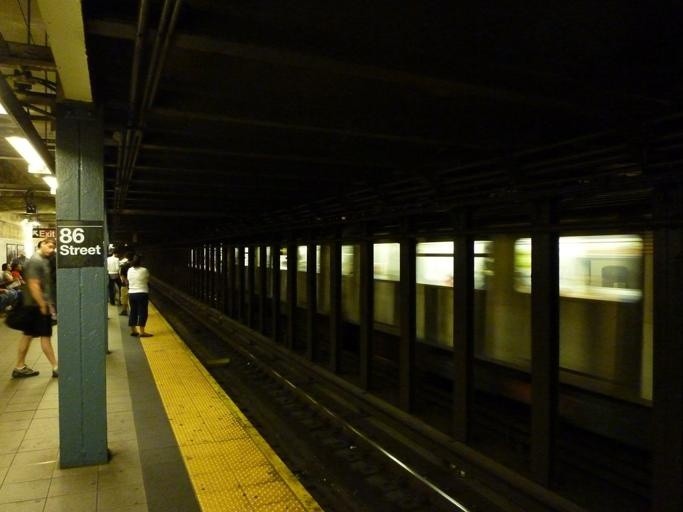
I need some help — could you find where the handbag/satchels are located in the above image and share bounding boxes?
[6,306,42,335]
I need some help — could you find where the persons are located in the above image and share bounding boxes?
[0,236,153,378]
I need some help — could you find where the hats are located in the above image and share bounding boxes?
[37,237,56,247]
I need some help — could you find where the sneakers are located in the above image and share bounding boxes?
[12,364,39,377]
[120,311,128,316]
[52,367,58,377]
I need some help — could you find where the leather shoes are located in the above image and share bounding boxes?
[131,332,139,336]
[140,332,152,337]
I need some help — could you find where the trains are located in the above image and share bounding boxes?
[144,128,681,510]
